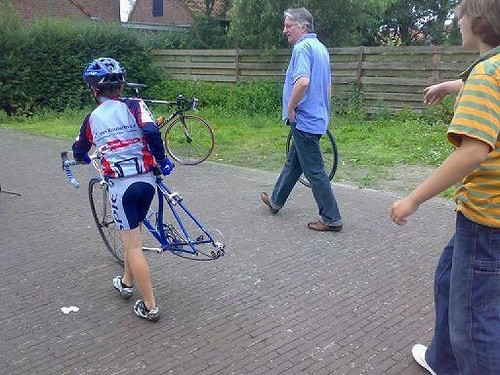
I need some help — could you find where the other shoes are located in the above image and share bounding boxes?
[261,192,279,214]
[412,343,437,375]
[308,221,343,231]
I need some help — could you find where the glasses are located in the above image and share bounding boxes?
[284,9,300,26]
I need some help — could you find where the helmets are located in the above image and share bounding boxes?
[83,57,126,86]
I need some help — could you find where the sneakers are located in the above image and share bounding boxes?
[113,275,134,299]
[134,298,161,322]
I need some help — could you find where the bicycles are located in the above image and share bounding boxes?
[61,148,226,267]
[118,84,214,165]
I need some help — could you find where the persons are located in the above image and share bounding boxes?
[390,1,500,375]
[260,7,344,232]
[72,57,173,323]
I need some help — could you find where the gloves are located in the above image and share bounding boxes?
[72,145,91,165]
[156,154,175,175]
